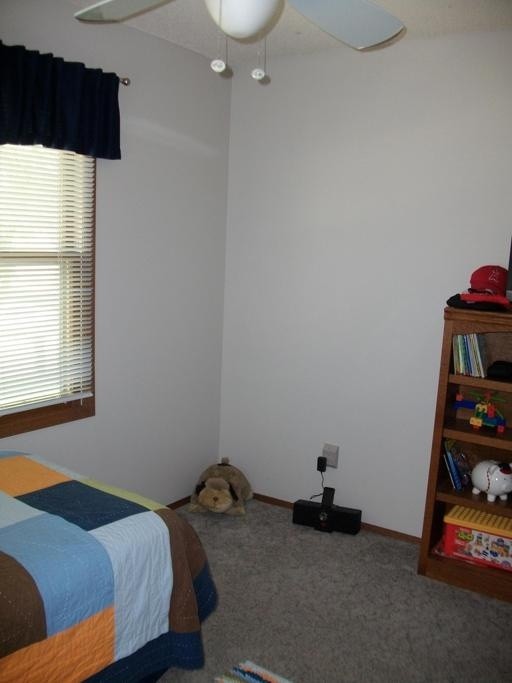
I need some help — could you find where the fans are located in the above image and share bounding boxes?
[74,0,408,89]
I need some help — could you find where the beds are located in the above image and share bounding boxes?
[0,447,194,683]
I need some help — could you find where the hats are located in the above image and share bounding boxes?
[460,265,509,304]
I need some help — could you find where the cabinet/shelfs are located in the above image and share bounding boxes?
[417,304,511,603]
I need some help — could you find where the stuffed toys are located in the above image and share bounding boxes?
[188,457,254,517]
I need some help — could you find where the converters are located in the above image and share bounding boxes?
[317,457,326,471]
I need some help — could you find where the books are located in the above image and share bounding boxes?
[453,333,489,380]
[442,439,473,493]
